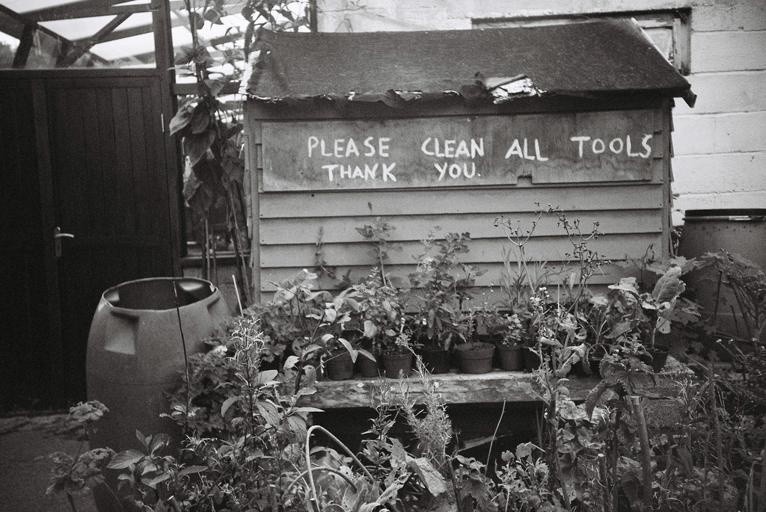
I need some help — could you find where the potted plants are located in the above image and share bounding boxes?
[272,202,703,382]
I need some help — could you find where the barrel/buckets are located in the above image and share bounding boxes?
[84,279,234,508]
[680,207,766,360]
